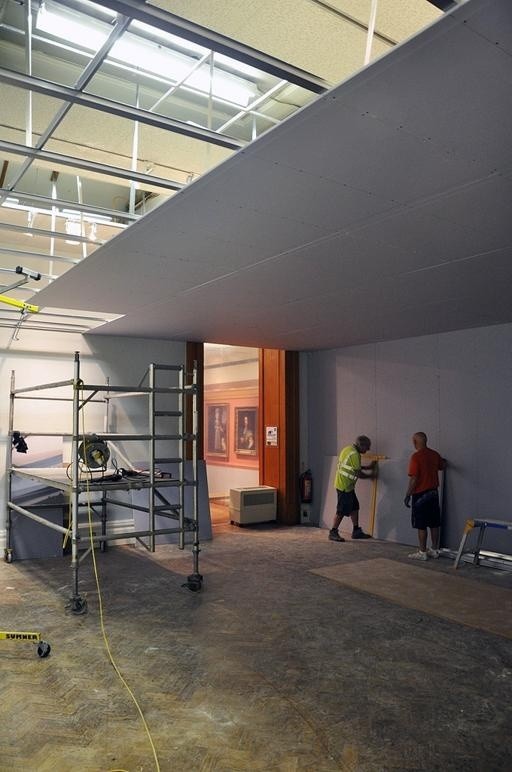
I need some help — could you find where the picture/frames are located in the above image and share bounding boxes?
[203,401,228,459]
[233,404,259,457]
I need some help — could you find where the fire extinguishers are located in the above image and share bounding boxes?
[298,469,313,503]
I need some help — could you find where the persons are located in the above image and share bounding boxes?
[240,417,253,449]
[209,408,225,452]
[328,436,376,541]
[404,432,444,561]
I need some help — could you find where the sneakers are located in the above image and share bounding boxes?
[427,548,440,559]
[328,530,346,542]
[351,527,372,539]
[408,550,427,561]
